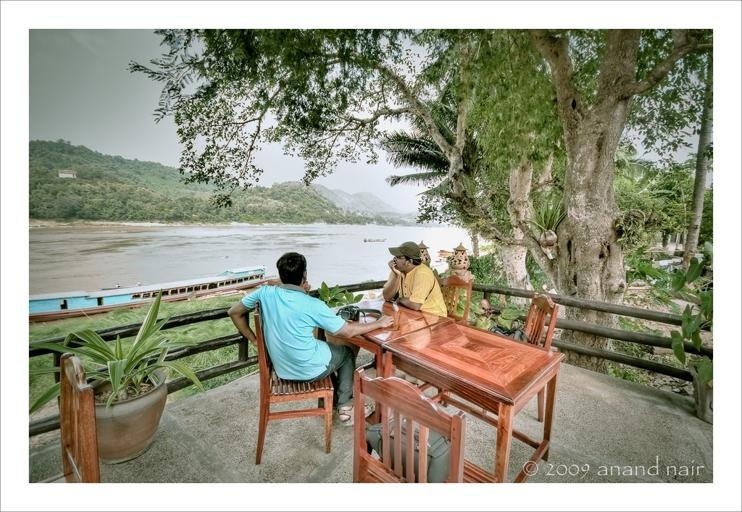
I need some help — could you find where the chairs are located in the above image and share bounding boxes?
[436,271,474,317]
[254,301,335,464]
[37,352,101,483]
[352,367,495,483]
[521,291,559,423]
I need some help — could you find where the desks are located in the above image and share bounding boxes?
[325,299,453,424]
[383,322,566,483]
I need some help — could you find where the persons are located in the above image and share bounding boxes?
[381,241,448,320]
[227,253,394,425]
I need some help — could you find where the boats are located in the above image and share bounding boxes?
[29,263,266,318]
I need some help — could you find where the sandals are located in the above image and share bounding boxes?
[338,397,376,426]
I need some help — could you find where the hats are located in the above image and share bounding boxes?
[388,241,421,261]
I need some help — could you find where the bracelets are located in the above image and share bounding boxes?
[399,298,400,304]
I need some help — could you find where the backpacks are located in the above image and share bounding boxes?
[362,412,451,483]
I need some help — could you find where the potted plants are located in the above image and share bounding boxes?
[527,194,567,247]
[673,245,713,424]
[29,287,202,466]
[316,283,363,363]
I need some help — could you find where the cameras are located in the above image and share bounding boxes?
[341,305,360,321]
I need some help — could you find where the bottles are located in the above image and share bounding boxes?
[391,302,400,331]
